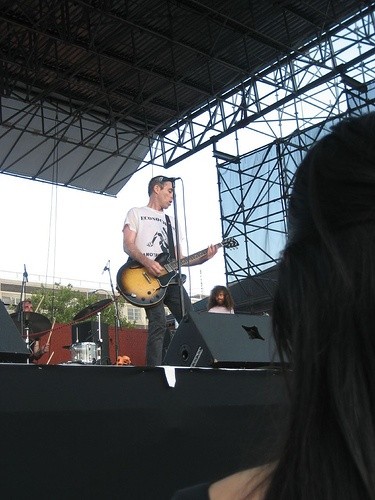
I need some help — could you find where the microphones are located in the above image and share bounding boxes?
[158,177,180,183]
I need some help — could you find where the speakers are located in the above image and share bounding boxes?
[71,320,111,365]
[0,299,31,363]
[161,310,290,370]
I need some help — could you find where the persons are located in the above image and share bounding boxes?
[208,285,235,313]
[174,113,375,500]
[17,301,50,362]
[122,176,218,366]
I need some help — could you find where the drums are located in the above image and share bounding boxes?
[70,342,99,364]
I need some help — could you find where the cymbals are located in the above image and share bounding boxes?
[9,311,52,341]
[72,299,112,322]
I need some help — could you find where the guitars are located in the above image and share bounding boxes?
[115,236,239,308]
